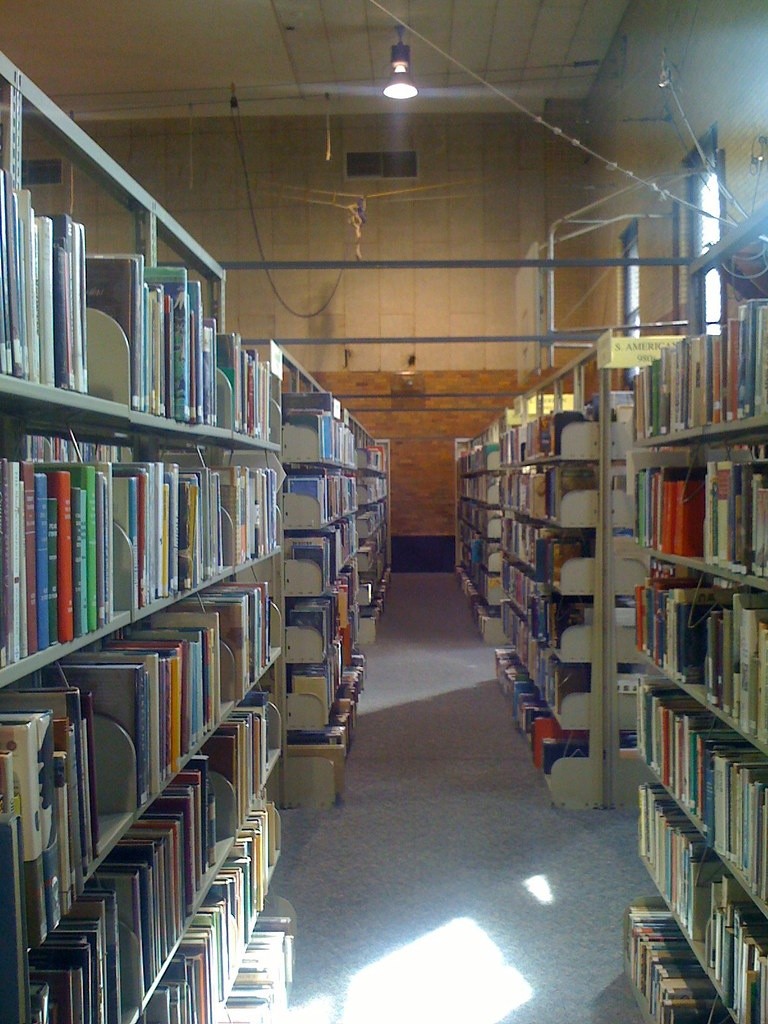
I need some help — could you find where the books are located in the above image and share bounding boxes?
[0,205,389,1024]
[453,297,768,1024]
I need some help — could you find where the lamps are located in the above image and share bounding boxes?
[381,27,420,99]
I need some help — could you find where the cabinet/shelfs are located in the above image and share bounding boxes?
[456,206,767,1023]
[0,52,388,1024]
